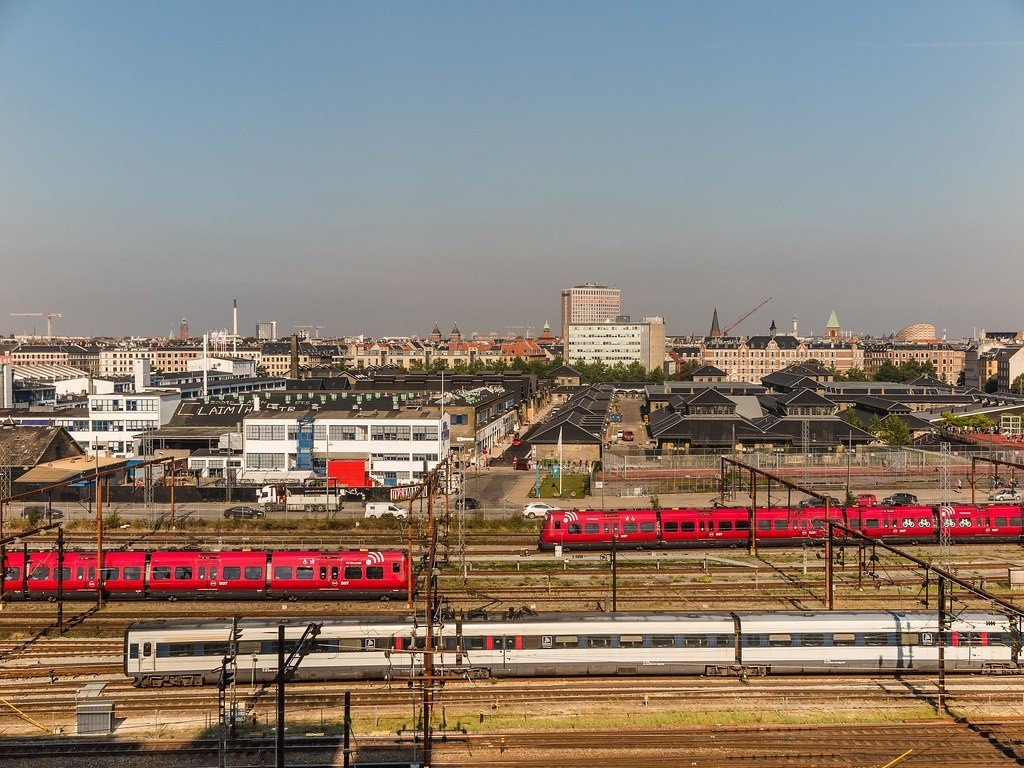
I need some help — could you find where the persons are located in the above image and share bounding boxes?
[947,424,1024,444]
[453,452,460,468]
[513,456,517,470]
[956,478,963,493]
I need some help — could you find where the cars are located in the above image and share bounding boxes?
[988,489,1022,501]
[799,495,840,506]
[21,506,63,519]
[135,477,145,485]
[882,493,918,505]
[224,506,266,520]
[617,430,624,437]
[515,458,539,470]
[551,408,560,417]
[852,494,877,505]
[455,498,480,510]
[512,439,522,446]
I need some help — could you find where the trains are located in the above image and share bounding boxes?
[125,615,1024,690]
[536,503,1024,552]
[1,549,417,601]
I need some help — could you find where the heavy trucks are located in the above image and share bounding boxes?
[257,485,342,513]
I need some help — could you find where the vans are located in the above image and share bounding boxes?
[611,414,622,423]
[622,430,635,441]
[365,502,408,519]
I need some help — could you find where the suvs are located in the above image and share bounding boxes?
[522,502,562,519]
